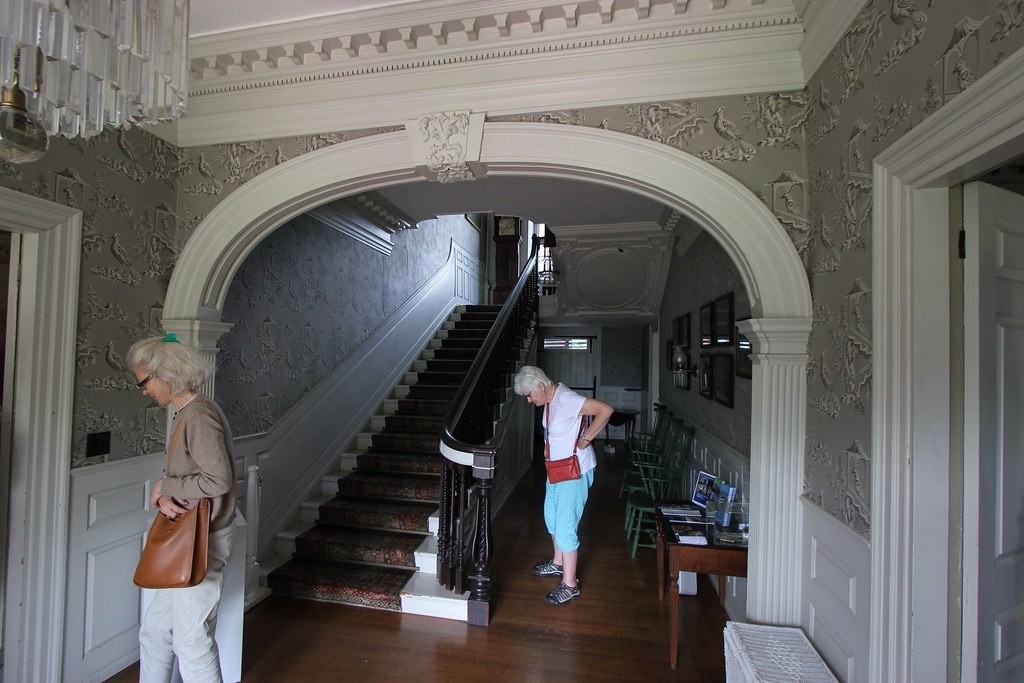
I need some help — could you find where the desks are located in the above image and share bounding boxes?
[606,408,640,443]
[655,499,748,672]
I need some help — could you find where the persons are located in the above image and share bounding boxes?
[127,334,246,683]
[513,366,615,607]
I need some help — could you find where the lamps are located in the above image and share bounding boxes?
[671,345,698,377]
[0,46,50,165]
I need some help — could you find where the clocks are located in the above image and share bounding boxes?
[498,217,516,235]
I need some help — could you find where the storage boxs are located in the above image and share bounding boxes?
[719,620,840,682]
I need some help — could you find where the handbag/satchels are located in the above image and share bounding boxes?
[132,416,211,590]
[544,454,581,484]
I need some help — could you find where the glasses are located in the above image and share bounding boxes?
[135,370,155,390]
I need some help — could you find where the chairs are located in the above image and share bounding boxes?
[617,403,696,558]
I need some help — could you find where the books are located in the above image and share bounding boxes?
[657,503,716,545]
[691,470,737,526]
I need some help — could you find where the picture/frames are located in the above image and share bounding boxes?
[666,292,740,409]
[690,470,718,508]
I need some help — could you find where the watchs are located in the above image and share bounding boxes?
[582,434,591,443]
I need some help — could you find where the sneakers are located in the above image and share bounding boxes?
[531,559,564,576]
[545,578,582,607]
